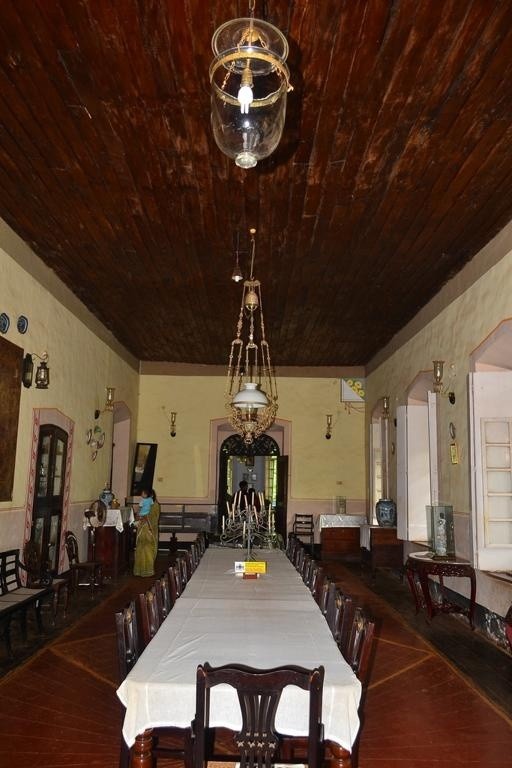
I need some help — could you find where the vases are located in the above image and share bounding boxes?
[376,499,396,526]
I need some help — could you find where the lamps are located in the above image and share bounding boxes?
[105,388,115,411]
[384,396,390,418]
[210,2,293,169]
[433,361,445,392]
[225,226,279,444]
[32,352,50,389]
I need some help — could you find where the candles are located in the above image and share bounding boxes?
[222,491,277,561]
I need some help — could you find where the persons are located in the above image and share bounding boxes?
[132,490,161,578]
[136,489,154,516]
[226,480,272,531]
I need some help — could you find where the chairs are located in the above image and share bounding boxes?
[115,535,376,768]
[293,513,314,554]
[0,531,102,665]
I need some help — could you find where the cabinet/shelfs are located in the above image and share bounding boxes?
[96,506,131,568]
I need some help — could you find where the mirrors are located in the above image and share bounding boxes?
[131,443,157,496]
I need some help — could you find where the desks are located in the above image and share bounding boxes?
[407,551,475,630]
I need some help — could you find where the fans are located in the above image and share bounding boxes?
[83,501,107,559]
